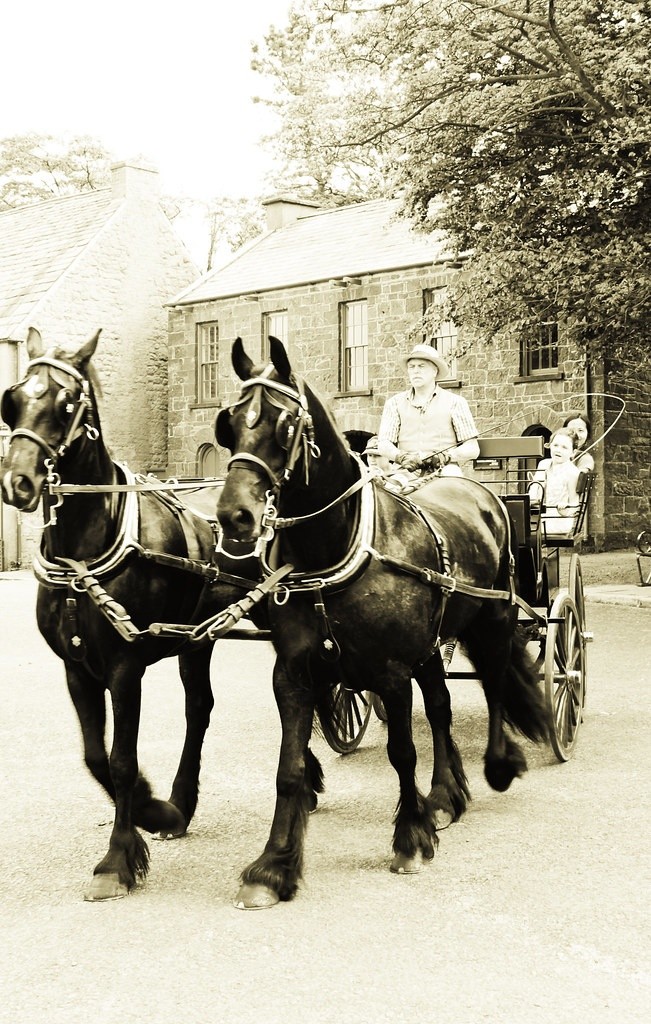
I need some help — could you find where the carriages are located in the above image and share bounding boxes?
[0,325,598,912]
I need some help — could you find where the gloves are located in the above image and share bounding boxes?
[421,449,451,468]
[395,450,426,472]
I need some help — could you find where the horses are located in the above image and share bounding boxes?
[213,335,552,912]
[0,324,247,902]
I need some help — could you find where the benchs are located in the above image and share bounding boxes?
[541,471,598,547]
[474,436,545,551]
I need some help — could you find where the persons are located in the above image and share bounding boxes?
[528,413,595,538]
[361,344,481,488]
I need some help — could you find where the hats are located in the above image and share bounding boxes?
[361,436,381,456]
[398,345,449,380]
